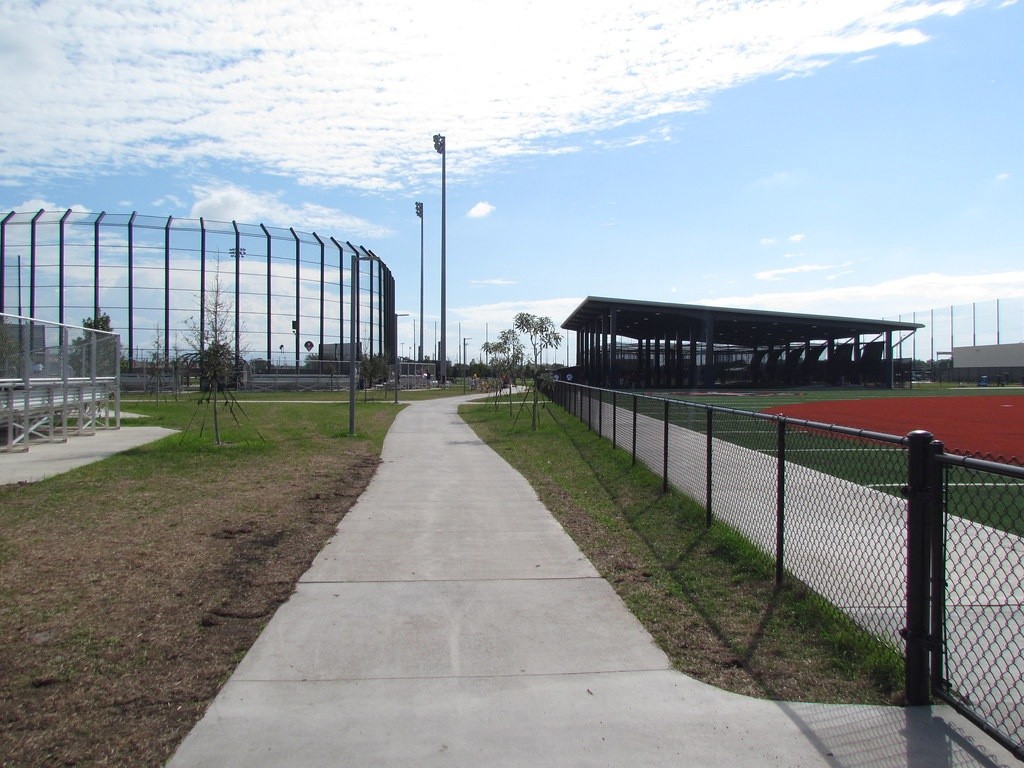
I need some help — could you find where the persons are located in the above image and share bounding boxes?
[425,370,431,389]
[567,372,572,383]
[360,377,365,390]
[67,361,73,377]
[145,376,151,390]
[471,370,477,391]
[33,360,44,373]
[8,361,17,378]
[554,373,559,380]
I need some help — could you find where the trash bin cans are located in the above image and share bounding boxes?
[359,379,366,389]
[200,376,210,392]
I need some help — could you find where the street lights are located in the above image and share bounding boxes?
[431,134,448,385]
[414,201,424,362]
